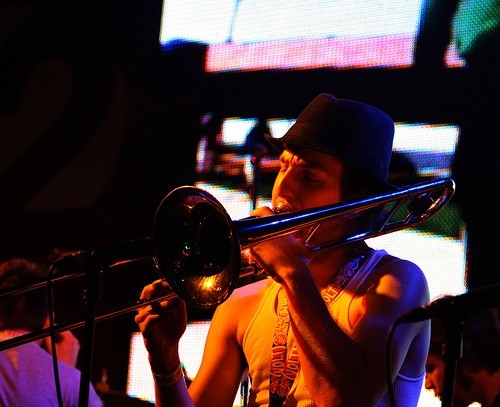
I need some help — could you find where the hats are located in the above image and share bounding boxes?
[264,93,413,204]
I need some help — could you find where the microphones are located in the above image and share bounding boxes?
[51,237,158,275]
[396,285,500,323]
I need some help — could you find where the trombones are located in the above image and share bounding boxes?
[0,177,455,351]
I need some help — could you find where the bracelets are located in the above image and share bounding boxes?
[151,363,183,387]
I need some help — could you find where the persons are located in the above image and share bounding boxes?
[0,258,105,406]
[425,292,500,407]
[134,93,431,407]
